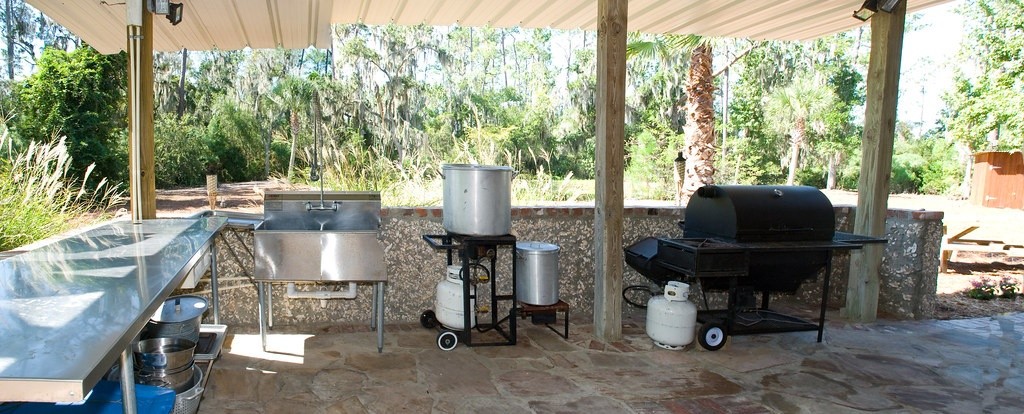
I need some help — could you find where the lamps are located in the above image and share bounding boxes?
[851,0,878,22]
[166,2,183,25]
[145,0,170,15]
[878,0,902,13]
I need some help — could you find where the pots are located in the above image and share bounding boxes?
[436,163,520,237]
[108,295,209,414]
[516,240,563,305]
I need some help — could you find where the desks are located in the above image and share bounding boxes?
[423,227,569,349]
